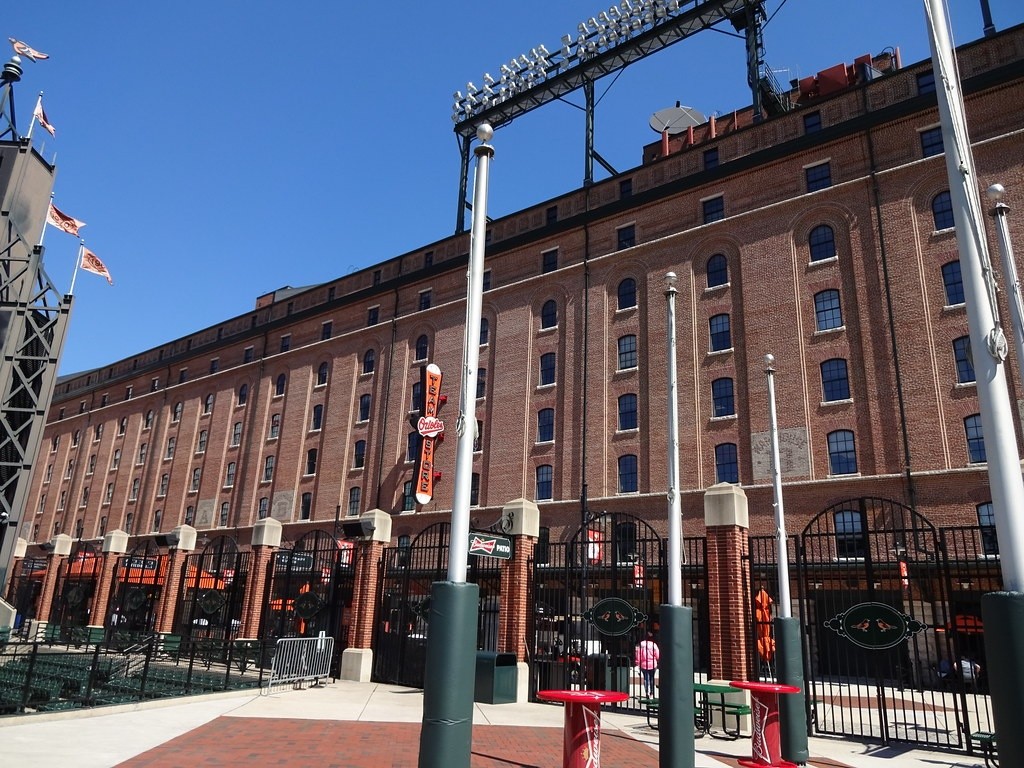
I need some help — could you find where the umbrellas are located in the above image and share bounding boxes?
[756,586,777,684]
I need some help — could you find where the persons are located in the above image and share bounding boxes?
[634,631,660,699]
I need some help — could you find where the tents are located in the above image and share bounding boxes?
[26,554,226,590]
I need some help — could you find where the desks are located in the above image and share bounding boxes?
[656,683,743,741]
[536,691,629,768]
[729,681,801,768]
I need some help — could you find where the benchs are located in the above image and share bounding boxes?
[638,698,658,704]
[725,709,751,715]
[698,700,750,708]
[646,704,702,714]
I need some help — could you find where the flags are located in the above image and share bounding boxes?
[46,205,87,238]
[81,246,113,285]
[35,102,56,138]
